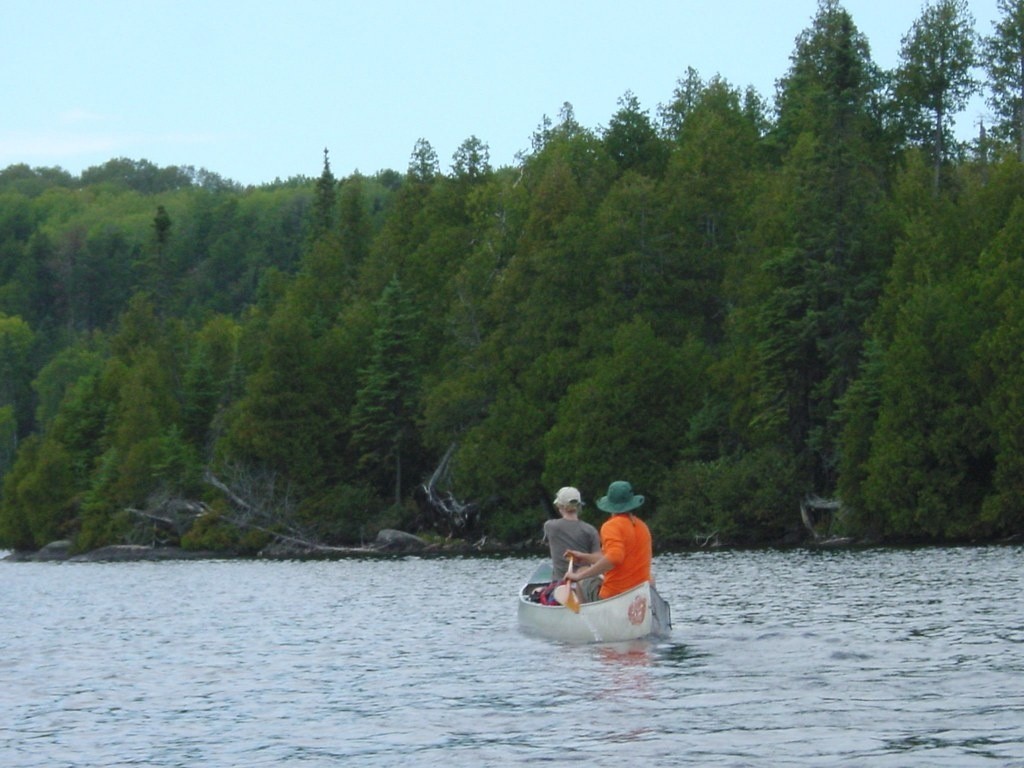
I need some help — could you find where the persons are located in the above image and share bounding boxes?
[531,487,599,606]
[562,480,652,604]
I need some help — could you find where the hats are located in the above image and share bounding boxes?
[596,480,644,513]
[553,487,581,508]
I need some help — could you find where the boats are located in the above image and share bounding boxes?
[514,557,674,641]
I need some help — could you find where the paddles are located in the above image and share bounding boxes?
[551,554,585,614]
